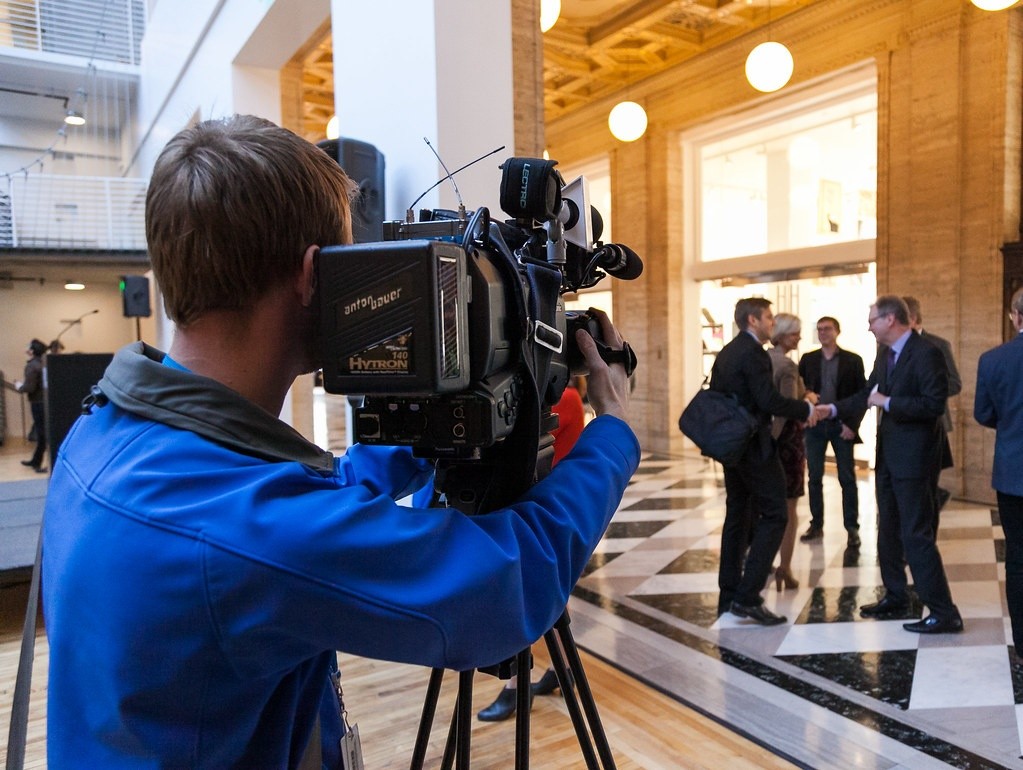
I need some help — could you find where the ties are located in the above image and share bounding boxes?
[885,347,897,386]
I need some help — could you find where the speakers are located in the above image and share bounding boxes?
[121,275,152,317]
[316,137,388,244]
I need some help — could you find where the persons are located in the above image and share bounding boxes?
[973,288,1023,656]
[798,316,868,547]
[47,340,64,354]
[43,111,641,770]
[476,383,583,722]
[764,312,818,594]
[709,297,819,626]
[14,339,48,468]
[815,295,964,633]
[902,295,962,565]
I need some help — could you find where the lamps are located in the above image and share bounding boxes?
[744,0,794,93]
[64,108,86,127]
[608,53,647,141]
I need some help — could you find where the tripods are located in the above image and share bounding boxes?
[407,433,617,770]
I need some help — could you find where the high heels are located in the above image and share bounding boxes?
[775,566,799,591]
[477,683,536,721]
[531,668,576,697]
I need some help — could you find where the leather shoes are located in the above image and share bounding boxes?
[903,612,962,634]
[718,602,729,618]
[731,600,787,626]
[860,597,909,619]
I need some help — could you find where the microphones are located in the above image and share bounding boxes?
[601,243,644,280]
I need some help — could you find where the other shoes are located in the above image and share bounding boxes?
[800,526,824,540]
[37,467,49,473]
[21,460,41,469]
[848,532,861,551]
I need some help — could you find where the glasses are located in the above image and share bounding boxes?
[1009,312,1016,320]
[869,313,888,325]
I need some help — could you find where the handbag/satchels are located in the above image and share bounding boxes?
[679,387,763,464]
